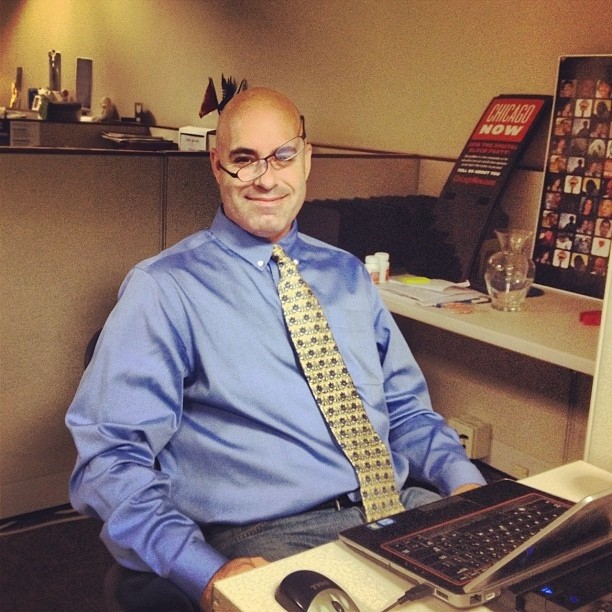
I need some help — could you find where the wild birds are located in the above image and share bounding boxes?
[338,477,611,608]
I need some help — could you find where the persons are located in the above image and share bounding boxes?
[533,78,612,279]
[63,88,488,611]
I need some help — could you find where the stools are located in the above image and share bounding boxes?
[308,487,362,511]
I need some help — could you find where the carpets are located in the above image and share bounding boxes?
[270,243,405,521]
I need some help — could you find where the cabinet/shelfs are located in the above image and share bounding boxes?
[364,256,380,283]
[375,252,390,281]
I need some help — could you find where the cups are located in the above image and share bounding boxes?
[217,116,306,182]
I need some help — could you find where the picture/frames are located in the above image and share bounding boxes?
[211,459,610,611]
[372,261,610,462]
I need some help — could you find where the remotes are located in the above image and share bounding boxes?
[274,569,360,612]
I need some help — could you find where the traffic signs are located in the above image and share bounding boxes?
[447,416,488,461]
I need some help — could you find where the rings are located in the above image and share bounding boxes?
[437,297,491,308]
